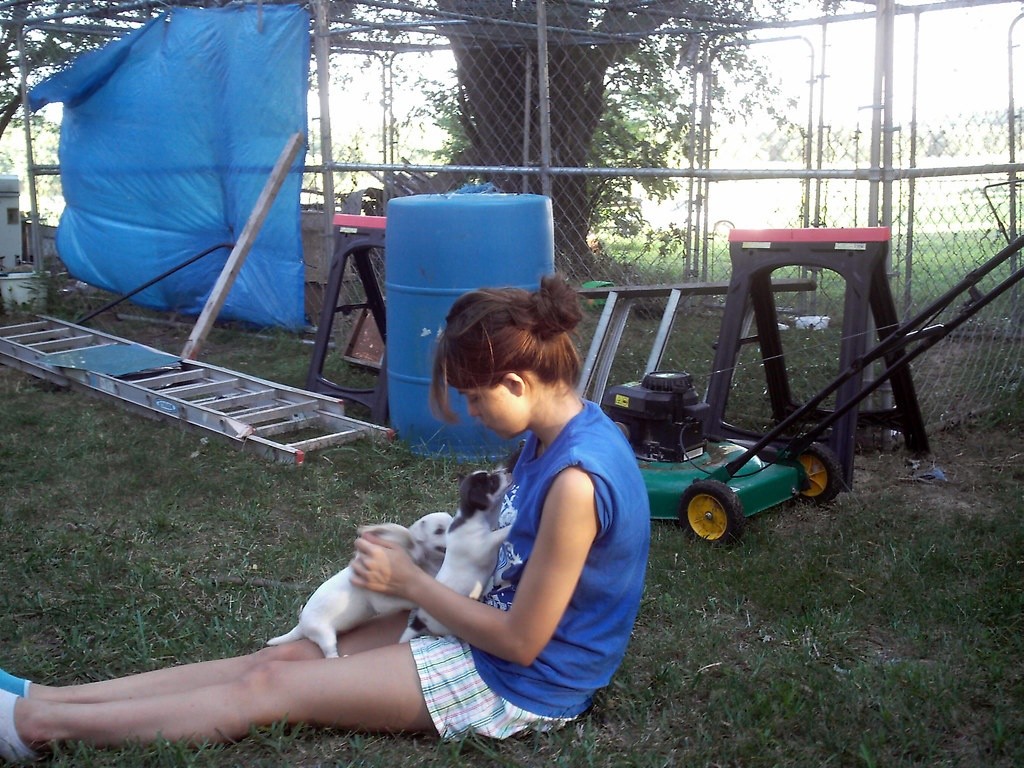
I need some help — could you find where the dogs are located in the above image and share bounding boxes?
[266,464,514,659]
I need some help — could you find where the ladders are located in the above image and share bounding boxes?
[1,310,397,466]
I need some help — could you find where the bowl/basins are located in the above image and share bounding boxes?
[795,316,830,329]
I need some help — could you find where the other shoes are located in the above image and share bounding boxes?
[1,669,31,700]
[0,689,38,761]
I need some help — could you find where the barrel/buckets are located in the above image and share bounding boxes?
[0,271,51,316]
[385,191,554,465]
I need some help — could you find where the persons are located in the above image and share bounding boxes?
[0,276,650,763]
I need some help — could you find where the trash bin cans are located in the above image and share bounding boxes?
[379,190,557,467]
